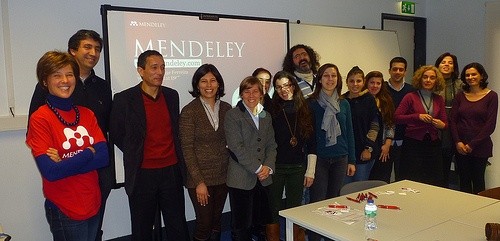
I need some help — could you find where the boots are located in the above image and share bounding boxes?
[266,223,280,241]
[293,225,306,241]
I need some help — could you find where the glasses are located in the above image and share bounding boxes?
[274,83,294,90]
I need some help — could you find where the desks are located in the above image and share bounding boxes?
[280,179,500,241]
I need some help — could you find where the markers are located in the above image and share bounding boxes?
[329,205,347,208]
[377,204,398,209]
[357,193,367,201]
[347,197,360,203]
[369,192,377,197]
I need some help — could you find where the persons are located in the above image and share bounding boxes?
[271,70,318,241]
[450,62,498,194]
[434,52,465,186]
[26,50,108,241]
[224,75,277,241]
[110,50,188,241]
[384,56,413,183]
[28,29,117,241]
[365,71,398,184]
[237,68,272,241]
[178,64,232,241]
[282,45,321,205]
[342,66,380,184]
[391,64,448,184]
[306,64,357,241]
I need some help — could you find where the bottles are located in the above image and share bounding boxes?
[364,200,377,231]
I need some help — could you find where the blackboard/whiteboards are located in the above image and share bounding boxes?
[289,22,406,94]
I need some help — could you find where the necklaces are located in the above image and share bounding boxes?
[281,106,297,147]
[46,100,79,126]
[248,108,257,116]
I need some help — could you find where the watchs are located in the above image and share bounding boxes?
[365,146,373,152]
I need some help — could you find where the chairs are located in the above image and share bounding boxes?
[340,181,387,197]
